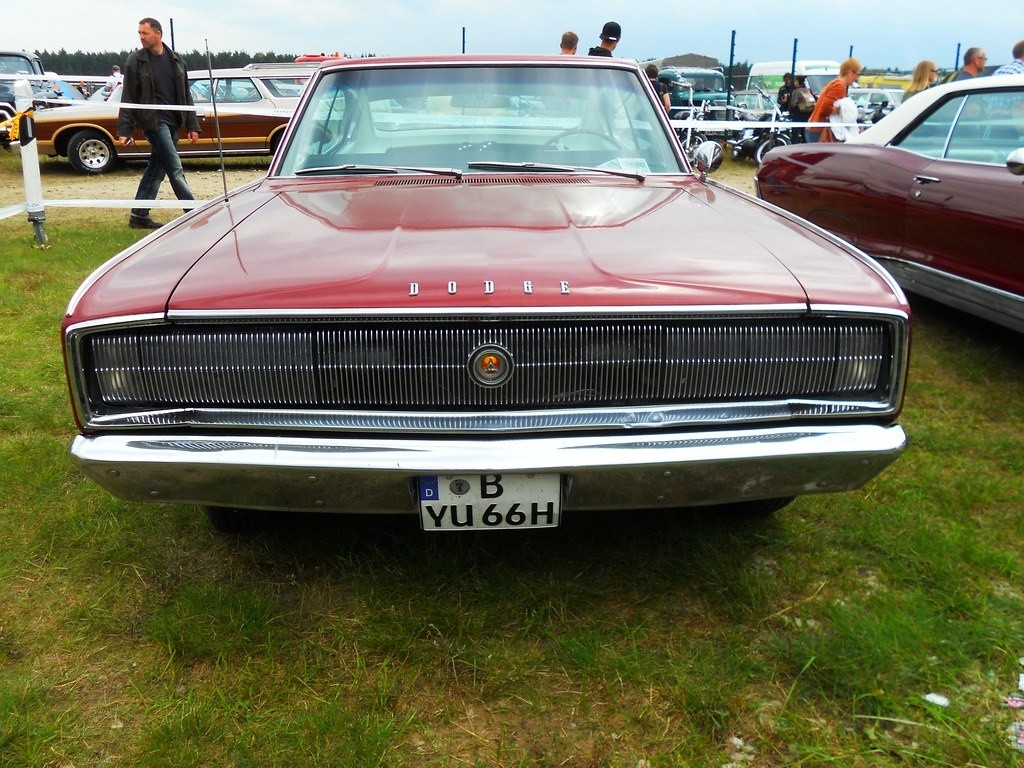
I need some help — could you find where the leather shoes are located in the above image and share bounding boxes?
[128,216,164,228]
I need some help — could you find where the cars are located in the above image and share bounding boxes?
[59,58,911,536]
[850,86,909,120]
[0,68,315,177]
[754,71,1024,340]
[0,52,45,122]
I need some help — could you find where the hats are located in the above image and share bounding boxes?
[599,21,620,41]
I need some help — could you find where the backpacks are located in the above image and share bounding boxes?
[796,88,817,113]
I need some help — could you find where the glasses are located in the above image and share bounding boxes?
[931,69,938,74]
[853,71,860,77]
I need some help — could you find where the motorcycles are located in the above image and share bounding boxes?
[675,81,713,170]
[725,85,789,166]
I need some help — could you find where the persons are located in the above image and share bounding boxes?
[790,75,818,144]
[105,64,125,94]
[116,17,203,226]
[101,86,114,101]
[991,39,1024,78]
[587,21,622,57]
[938,46,987,85]
[805,57,862,144]
[900,59,938,105]
[645,63,671,113]
[558,31,579,56]
[776,72,796,114]
[78,80,89,100]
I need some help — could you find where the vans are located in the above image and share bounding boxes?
[747,59,844,100]
[658,64,731,105]
